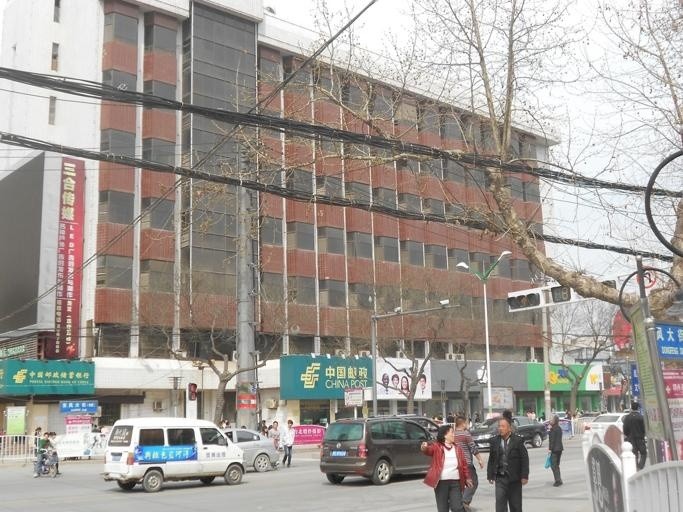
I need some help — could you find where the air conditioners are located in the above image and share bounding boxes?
[266,399,276,409]
[154,400,167,410]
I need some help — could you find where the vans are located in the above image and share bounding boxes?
[319,416,443,485]
[100,416,245,494]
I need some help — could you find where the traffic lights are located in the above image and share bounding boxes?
[598,279,616,289]
[188,383,197,401]
[507,293,540,310]
[550,285,571,303]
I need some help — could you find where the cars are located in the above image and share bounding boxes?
[406,414,442,439]
[468,416,546,452]
[583,412,631,435]
[209,428,279,473]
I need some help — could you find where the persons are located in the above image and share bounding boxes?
[380,372,390,392]
[280,419,296,465]
[418,375,427,396]
[491,409,519,438]
[487,418,529,512]
[420,425,473,512]
[34,427,60,479]
[432,411,483,423]
[401,376,409,393]
[526,405,584,421]
[268,421,280,452]
[546,414,563,487]
[623,401,647,471]
[218,418,272,437]
[453,416,484,512]
[389,374,399,395]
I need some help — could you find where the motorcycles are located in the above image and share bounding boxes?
[32,450,59,479]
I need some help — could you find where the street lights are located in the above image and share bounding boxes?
[453,249,514,413]
